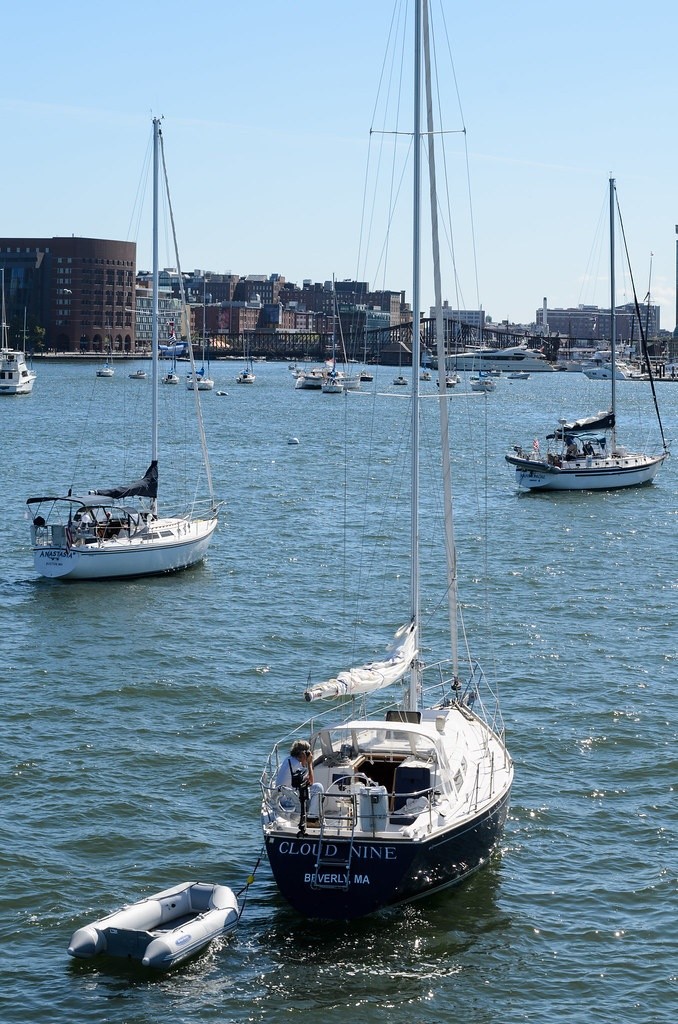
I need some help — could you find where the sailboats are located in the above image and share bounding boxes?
[469,304,503,392]
[234,307,256,385]
[185,275,215,391]
[419,328,433,381]
[287,271,374,394]
[504,167,677,496]
[435,313,462,389]
[0,263,39,396]
[392,320,409,386]
[259,0,518,928]
[25,107,227,585]
[161,323,180,385]
[96,315,117,377]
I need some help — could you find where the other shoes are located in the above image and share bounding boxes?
[307,820,320,828]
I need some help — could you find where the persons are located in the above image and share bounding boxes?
[274,738,323,827]
[582,444,596,456]
[553,456,561,468]
[566,451,578,461]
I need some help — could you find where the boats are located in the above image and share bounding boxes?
[561,349,678,384]
[127,369,147,379]
[506,367,531,380]
[67,880,241,975]
[420,336,561,373]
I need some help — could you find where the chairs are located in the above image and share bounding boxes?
[385,710,421,740]
